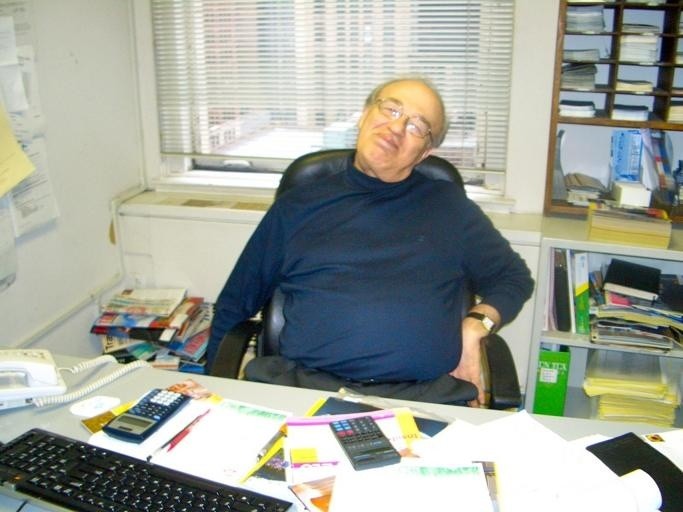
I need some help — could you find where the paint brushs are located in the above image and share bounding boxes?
[167,429,192,453]
[145,410,212,461]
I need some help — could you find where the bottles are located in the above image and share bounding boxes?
[673,160,683,212]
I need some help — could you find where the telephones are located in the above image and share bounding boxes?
[0,349,67,410]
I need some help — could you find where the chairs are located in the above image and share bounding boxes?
[211,146,523,412]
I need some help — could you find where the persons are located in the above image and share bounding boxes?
[204,76,532,411]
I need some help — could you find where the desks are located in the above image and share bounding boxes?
[0,356,683,512]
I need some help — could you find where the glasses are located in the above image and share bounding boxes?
[375,97,434,144]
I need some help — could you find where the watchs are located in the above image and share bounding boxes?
[466,312,495,335]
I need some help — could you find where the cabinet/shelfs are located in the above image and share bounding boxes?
[543,0,683,226]
[525,237,683,427]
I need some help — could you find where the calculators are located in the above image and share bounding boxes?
[329,416,401,471]
[102,389,193,444]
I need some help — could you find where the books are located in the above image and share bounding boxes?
[584,347,682,425]
[559,0,682,122]
[88,375,683,512]
[543,249,682,350]
[564,133,672,249]
[88,288,216,373]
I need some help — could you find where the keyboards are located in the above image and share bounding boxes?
[0,428,294,512]
